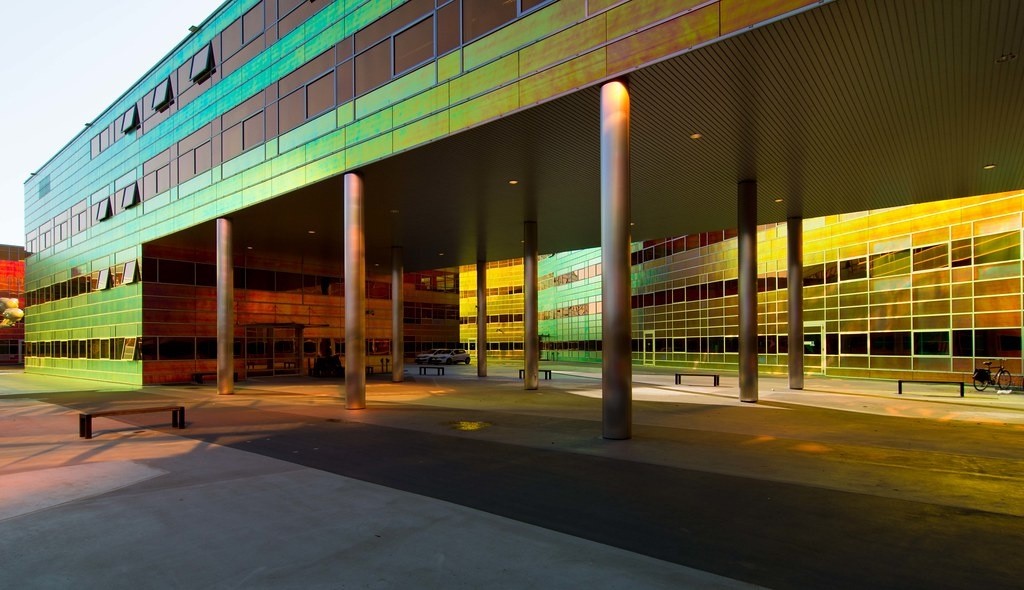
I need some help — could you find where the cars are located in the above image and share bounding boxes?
[416,350,440,364]
[430,349,472,365]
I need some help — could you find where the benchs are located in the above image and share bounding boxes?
[675,373,719,386]
[898,379,964,397]
[191,371,239,384]
[519,369,551,381]
[419,366,444,375]
[79,405,184,440]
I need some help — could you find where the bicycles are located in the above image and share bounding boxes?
[972,357,1013,391]
